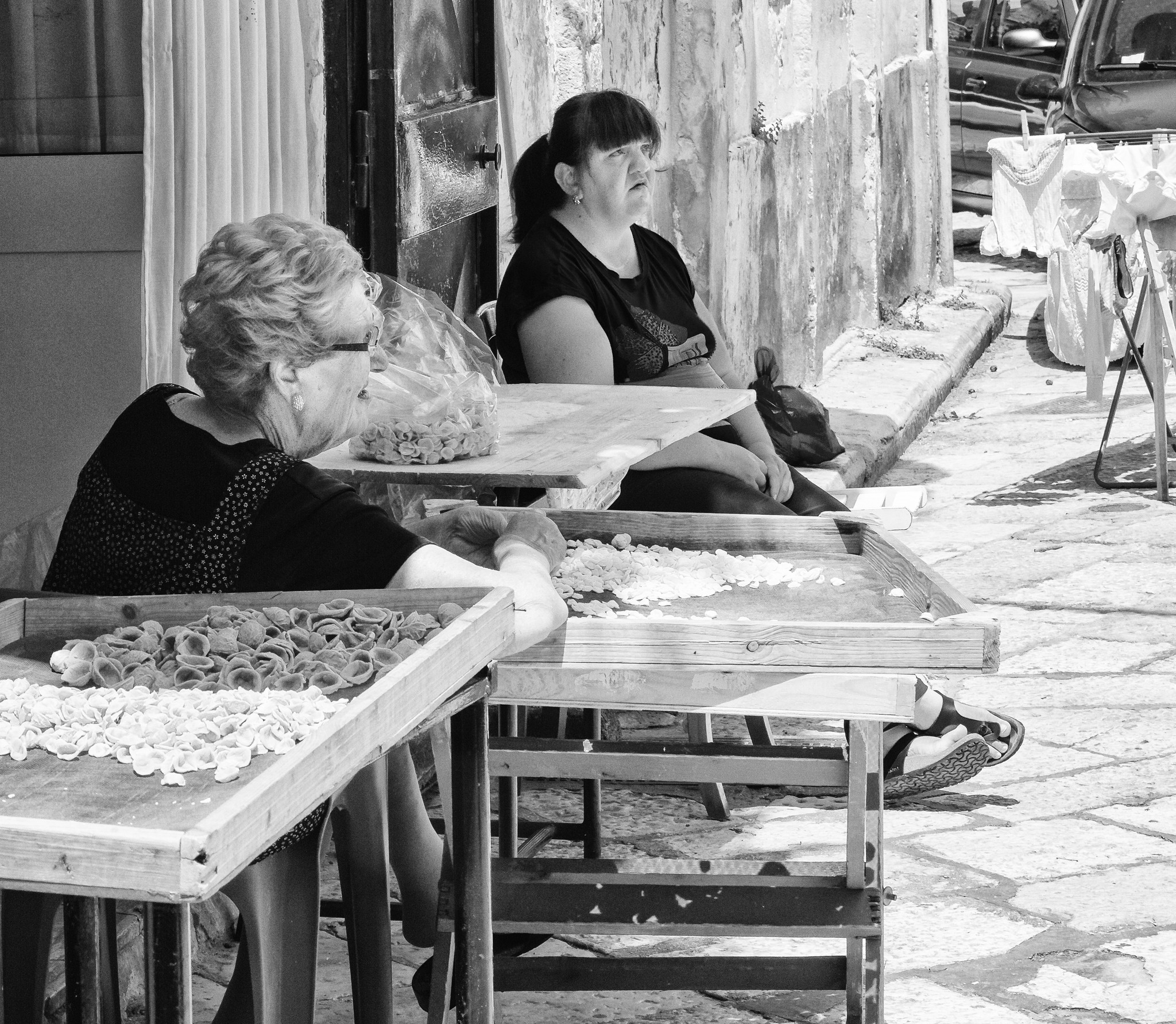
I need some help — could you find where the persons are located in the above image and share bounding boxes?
[495,92,1024,801]
[36,213,568,1017]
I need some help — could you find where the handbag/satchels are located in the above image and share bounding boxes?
[746,344,846,467]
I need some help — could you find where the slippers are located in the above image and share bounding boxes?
[407,932,556,1012]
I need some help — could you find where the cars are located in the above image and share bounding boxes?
[950,1,1176,213]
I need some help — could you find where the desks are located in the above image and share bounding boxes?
[0,384,920,1024]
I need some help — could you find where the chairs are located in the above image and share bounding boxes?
[1131,13,1176,60]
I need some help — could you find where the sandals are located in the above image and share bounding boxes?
[882,730,991,803]
[912,689,1025,767]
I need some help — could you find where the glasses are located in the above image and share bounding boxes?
[312,311,381,357]
